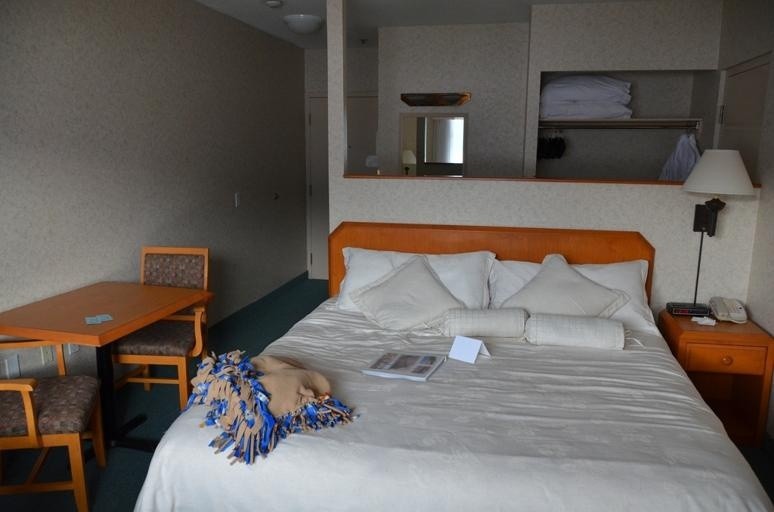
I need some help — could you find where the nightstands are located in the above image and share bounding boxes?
[657,309,774,449]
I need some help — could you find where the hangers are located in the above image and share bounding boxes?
[539,128,560,140]
[680,126,688,144]
[689,125,695,141]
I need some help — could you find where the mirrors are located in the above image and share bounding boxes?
[401,113,468,177]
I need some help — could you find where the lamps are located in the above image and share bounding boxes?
[283,13,322,35]
[663,148,756,317]
[265,0,281,8]
[402,148,417,176]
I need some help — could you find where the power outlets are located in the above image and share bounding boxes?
[40,346,53,365]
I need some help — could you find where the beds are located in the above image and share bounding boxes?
[131,221,774,512]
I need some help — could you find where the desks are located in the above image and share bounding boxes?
[0,281,213,465]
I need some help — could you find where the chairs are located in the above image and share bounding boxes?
[0,340,106,512]
[112,246,209,412]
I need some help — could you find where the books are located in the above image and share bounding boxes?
[362,349,446,381]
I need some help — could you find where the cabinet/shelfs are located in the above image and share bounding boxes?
[535,68,717,180]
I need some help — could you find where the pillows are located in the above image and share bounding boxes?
[498,253,631,316]
[441,308,529,338]
[336,246,496,316]
[489,259,661,334]
[349,253,467,333]
[524,313,625,349]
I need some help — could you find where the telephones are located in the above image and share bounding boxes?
[709,297,748,324]
